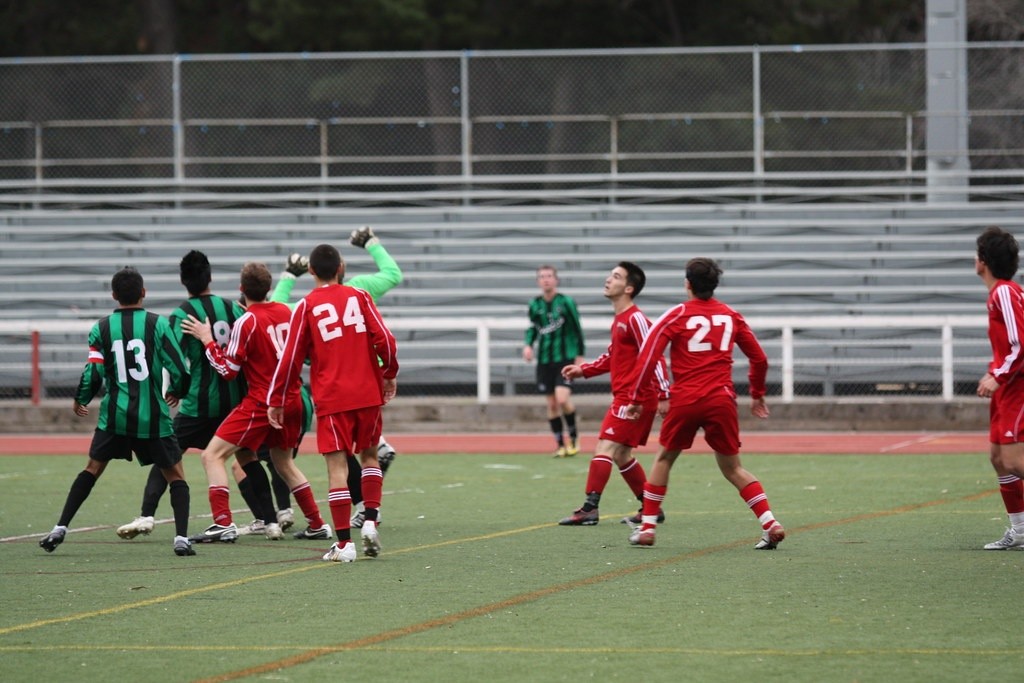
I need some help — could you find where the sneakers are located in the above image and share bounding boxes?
[39,524,69,552]
[567,436,580,456]
[322,541,357,563]
[621,507,666,523]
[376,443,397,478]
[362,533,380,557]
[173,535,192,556]
[264,522,285,540]
[188,523,239,543]
[982,527,1024,551]
[238,518,268,535]
[350,508,383,528]
[292,524,333,540]
[627,526,657,547]
[754,521,786,550]
[276,508,295,532]
[554,448,566,458]
[558,506,598,525]
[116,516,155,540]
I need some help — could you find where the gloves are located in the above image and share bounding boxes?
[349,226,373,247]
[286,252,309,275]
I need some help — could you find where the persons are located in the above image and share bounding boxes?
[40,266,196,557]
[560,261,670,526]
[625,258,785,549]
[266,244,399,562]
[523,267,585,457]
[115,223,404,542]
[975,226,1024,551]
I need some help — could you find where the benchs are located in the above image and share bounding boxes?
[0,167,1023,387]
